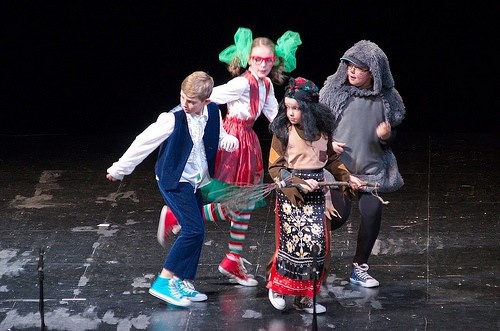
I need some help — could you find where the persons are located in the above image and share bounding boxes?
[319,40,407,286]
[158,27,301,286]
[106,72,239,306]
[268,78,367,313]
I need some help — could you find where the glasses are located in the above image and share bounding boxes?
[348,64,369,72]
[250,55,276,67]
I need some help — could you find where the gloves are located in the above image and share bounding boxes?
[220,135,239,152]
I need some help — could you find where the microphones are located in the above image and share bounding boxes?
[312,245,319,281]
[38,246,45,279]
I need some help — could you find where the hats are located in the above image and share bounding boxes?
[340,57,367,68]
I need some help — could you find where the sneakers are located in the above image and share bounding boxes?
[292,295,326,314]
[148,271,192,308]
[268,288,287,310]
[350,262,380,288]
[157,205,181,247]
[175,278,208,302]
[218,252,258,287]
